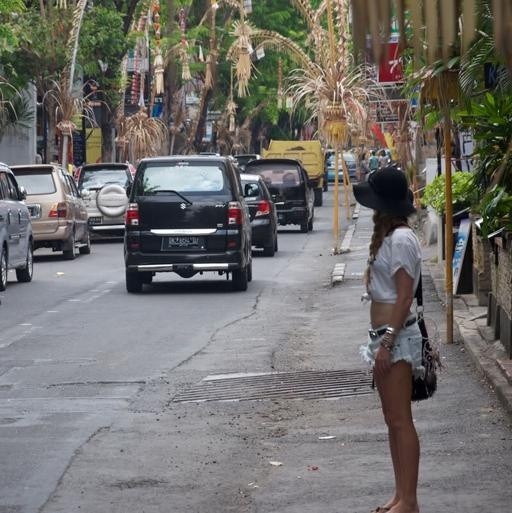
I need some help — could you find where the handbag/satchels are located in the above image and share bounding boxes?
[412,318,437,400]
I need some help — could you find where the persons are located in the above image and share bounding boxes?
[360,153,371,174]
[379,150,388,168]
[368,151,379,171]
[352,166,426,513]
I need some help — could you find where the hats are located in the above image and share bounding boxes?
[350,167,416,215]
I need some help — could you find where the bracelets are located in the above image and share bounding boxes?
[380,326,400,352]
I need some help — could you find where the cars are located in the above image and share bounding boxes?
[326,149,356,181]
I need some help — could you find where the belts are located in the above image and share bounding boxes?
[370,317,416,339]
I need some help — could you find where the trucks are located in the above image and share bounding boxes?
[260,139,328,206]
